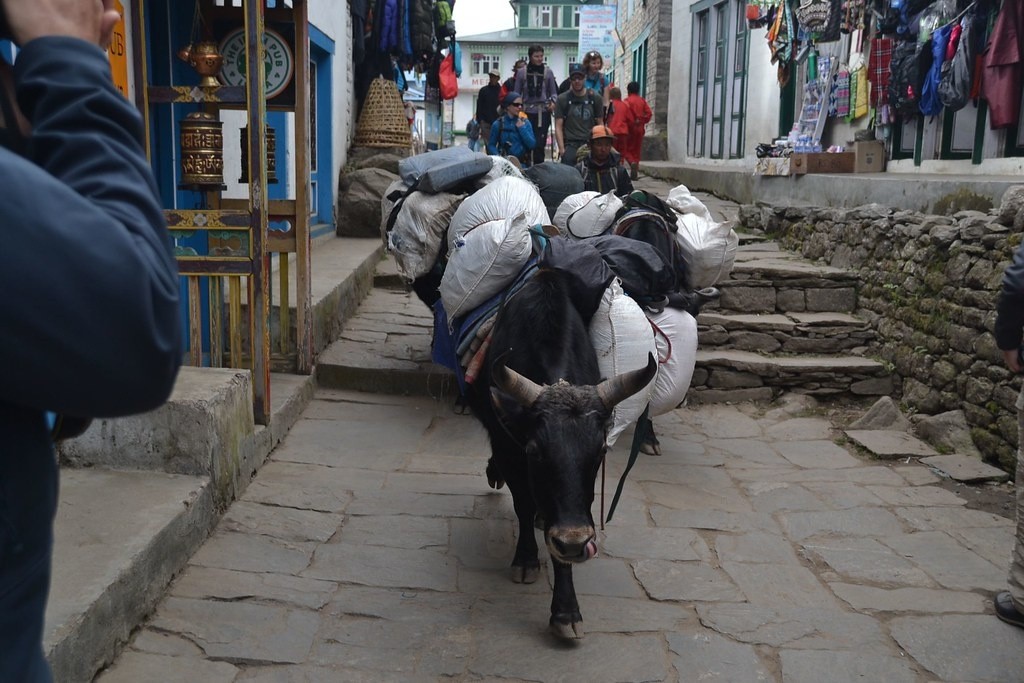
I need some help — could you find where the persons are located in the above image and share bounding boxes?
[0,0,182,683]
[991,249,1023,629]
[466,44,654,197]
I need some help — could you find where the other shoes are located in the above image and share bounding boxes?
[995,591,1024,627]
[631,162,637,180]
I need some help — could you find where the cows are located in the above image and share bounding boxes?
[381,177,661,645]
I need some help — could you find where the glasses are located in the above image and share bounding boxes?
[512,102,523,107]
[589,52,599,58]
[571,76,583,81]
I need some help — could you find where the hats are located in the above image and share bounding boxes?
[570,64,585,76]
[488,69,500,80]
[502,92,521,108]
[591,125,618,141]
